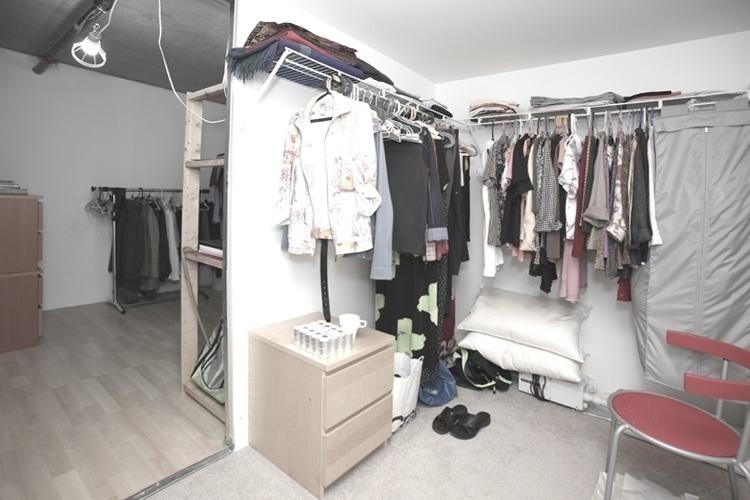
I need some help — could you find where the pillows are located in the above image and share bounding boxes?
[456,284,594,385]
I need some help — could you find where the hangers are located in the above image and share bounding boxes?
[484,106,655,156]
[290,69,479,158]
[84,184,210,219]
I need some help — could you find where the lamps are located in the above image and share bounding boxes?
[68,23,109,71]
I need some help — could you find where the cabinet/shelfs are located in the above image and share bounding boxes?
[0,193,46,354]
[247,309,399,498]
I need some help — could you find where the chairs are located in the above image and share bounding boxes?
[603,329,750,500]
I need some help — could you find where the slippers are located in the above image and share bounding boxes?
[433,404,467,434]
[450,411,490,439]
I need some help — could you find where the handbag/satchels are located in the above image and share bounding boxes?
[446,346,513,393]
[392,355,425,434]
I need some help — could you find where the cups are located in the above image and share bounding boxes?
[338,314,367,343]
